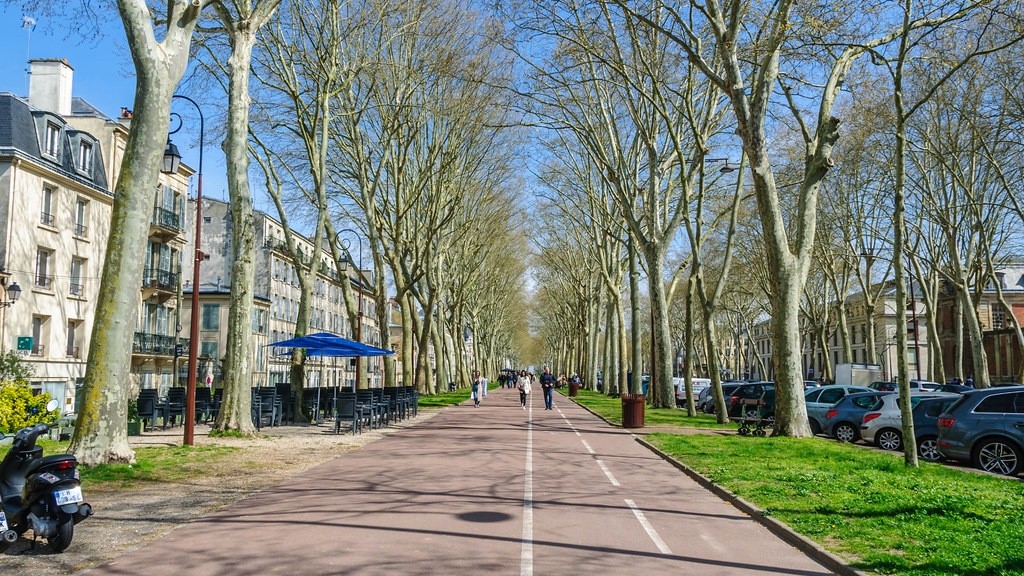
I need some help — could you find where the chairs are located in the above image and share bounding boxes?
[136,382,418,436]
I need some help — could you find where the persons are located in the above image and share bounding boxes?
[497,369,536,389]
[539,366,557,410]
[472,370,483,408]
[517,370,532,410]
[951,377,973,387]
[557,372,581,389]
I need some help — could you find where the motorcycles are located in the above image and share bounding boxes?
[0,400,94,553]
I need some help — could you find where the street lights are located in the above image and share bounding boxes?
[333,227,364,389]
[651,157,736,405]
[160,98,209,445]
[861,246,925,380]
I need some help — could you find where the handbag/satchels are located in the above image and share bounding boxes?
[472,384,478,392]
[519,385,524,391]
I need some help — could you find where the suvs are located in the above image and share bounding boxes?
[728,382,775,422]
[936,385,1023,474]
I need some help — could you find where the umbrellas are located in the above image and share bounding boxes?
[262,331,396,426]
[500,368,516,374]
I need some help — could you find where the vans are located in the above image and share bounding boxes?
[677,377,725,408]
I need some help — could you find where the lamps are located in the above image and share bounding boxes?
[0,282,21,309]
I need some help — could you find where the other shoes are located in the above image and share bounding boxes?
[520,406,526,410]
[545,407,548,410]
[474,404,479,408]
[549,407,552,410]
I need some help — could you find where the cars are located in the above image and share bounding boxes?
[910,380,942,393]
[933,385,975,392]
[698,379,757,414]
[642,376,681,395]
[804,385,879,435]
[823,391,900,442]
[868,382,899,391]
[912,396,968,461]
[860,392,962,451]
[804,380,821,389]
[757,385,777,428]
